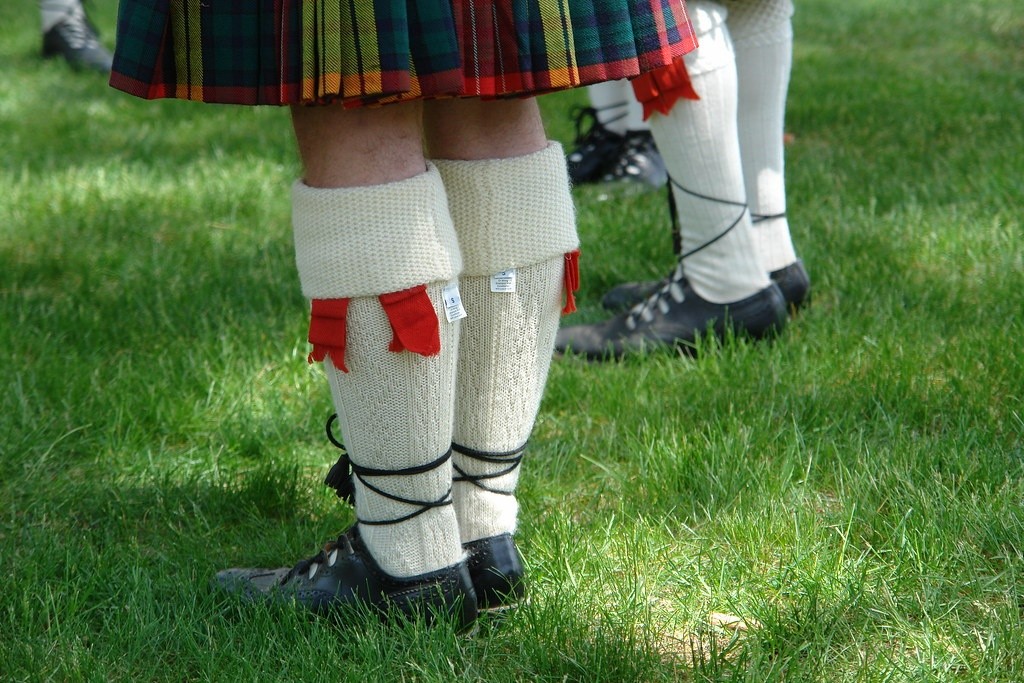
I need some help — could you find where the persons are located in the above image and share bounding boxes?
[108,0,700,635]
[39,0,113,75]
[554,0,812,364]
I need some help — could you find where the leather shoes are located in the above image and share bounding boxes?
[461,531,524,614]
[565,104,668,198]
[554,274,788,361]
[207,520,478,643]
[42,17,112,78]
[602,259,810,322]
